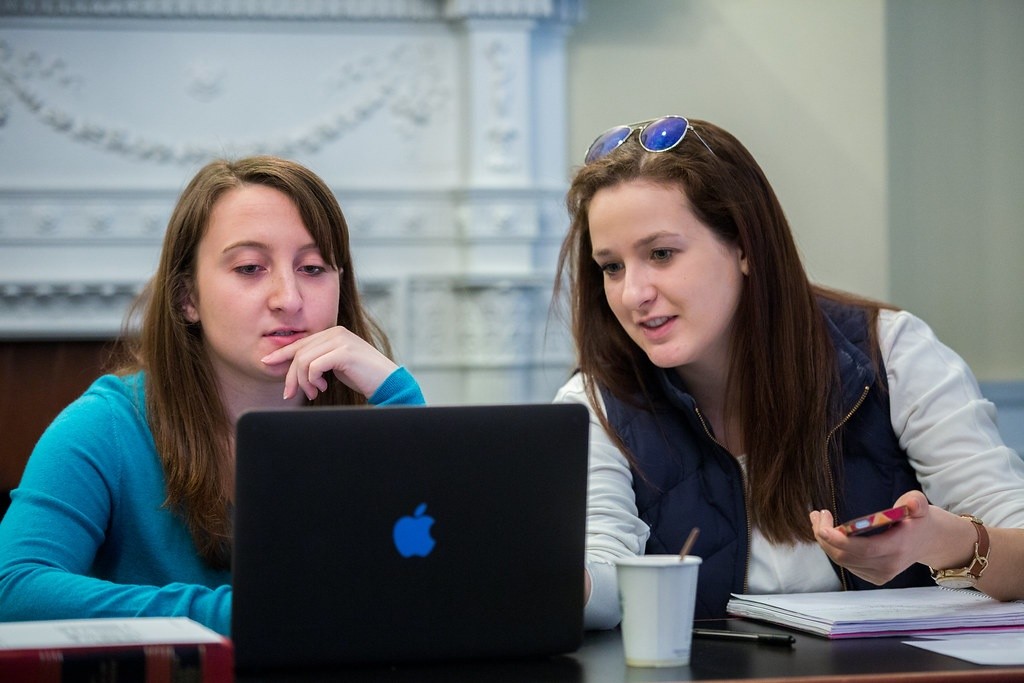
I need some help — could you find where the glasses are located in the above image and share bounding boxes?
[583,115,720,168]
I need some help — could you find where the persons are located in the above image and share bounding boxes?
[551,117,1024,631]
[0,155,427,640]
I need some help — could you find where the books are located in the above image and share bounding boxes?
[0,615,234,683]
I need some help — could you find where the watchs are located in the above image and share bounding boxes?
[928,514,990,589]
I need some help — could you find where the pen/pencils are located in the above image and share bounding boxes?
[692,629,796,646]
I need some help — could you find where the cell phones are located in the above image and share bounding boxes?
[832,505,911,535]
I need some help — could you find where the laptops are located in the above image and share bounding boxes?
[231,401,590,683]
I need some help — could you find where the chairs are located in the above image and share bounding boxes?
[0,336,139,526]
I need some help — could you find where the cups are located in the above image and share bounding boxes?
[615,555,701,668]
